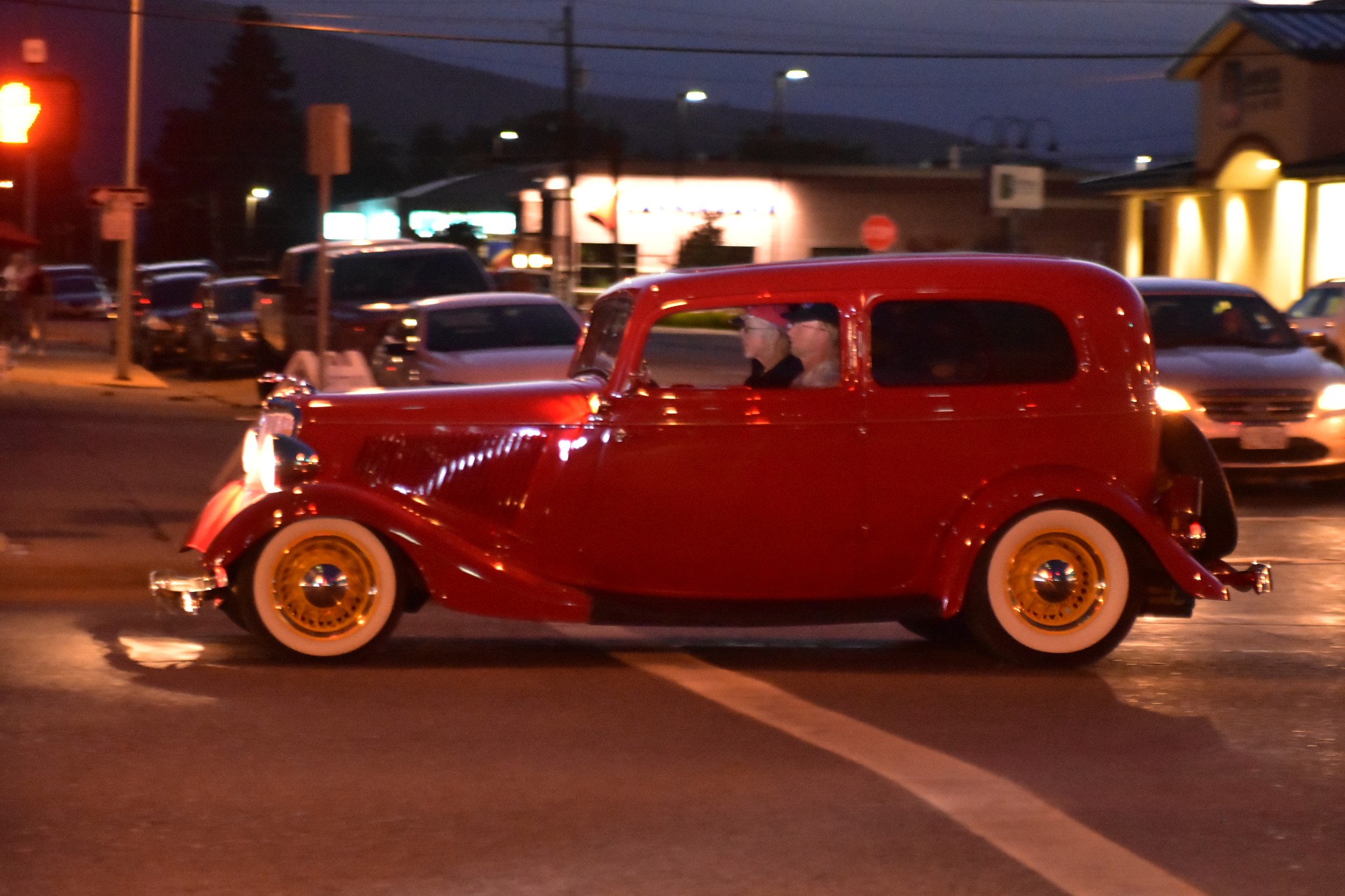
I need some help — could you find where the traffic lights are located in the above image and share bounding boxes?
[0,76,50,151]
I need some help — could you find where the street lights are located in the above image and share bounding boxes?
[244,187,270,232]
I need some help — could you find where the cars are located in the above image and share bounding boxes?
[367,292,586,386]
[1125,275,1345,491]
[27,237,489,381]
[1285,276,1345,368]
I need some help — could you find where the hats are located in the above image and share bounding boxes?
[741,302,792,329]
[780,301,839,326]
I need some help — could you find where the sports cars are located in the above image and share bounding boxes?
[147,251,1274,665]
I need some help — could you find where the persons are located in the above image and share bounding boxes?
[1,252,54,357]
[787,302,842,390]
[739,305,803,389]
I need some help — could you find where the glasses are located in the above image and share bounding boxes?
[744,324,778,332]
[786,319,826,333]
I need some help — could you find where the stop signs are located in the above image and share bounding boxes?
[859,214,899,252]
